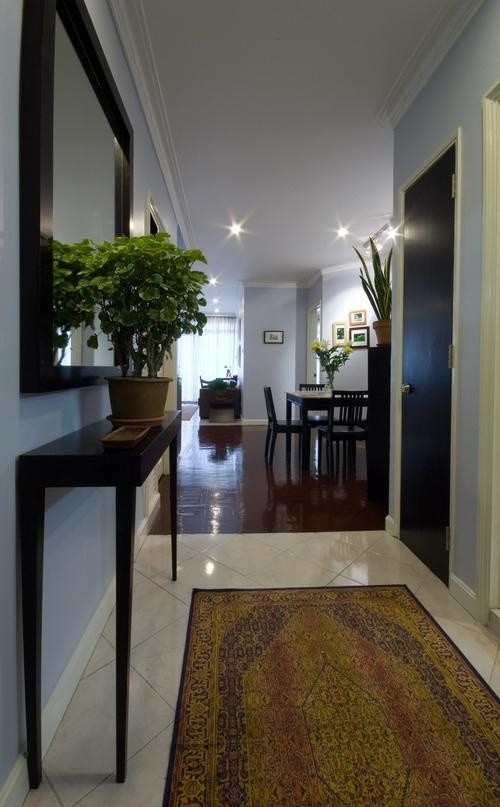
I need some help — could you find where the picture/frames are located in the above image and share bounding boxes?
[264,331,284,344]
[332,322,349,347]
[349,326,369,348]
[349,310,367,325]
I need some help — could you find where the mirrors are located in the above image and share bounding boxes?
[19,0,134,394]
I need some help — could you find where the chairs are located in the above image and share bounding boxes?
[263,384,368,482]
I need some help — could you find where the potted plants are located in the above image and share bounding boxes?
[53,234,207,429]
[351,237,393,347]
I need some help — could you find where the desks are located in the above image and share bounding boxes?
[15,411,180,788]
[198,388,241,420]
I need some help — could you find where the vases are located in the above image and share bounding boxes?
[322,386,334,395]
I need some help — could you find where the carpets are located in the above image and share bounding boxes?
[163,584,500,807]
[181,406,197,421]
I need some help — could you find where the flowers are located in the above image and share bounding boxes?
[311,338,353,388]
